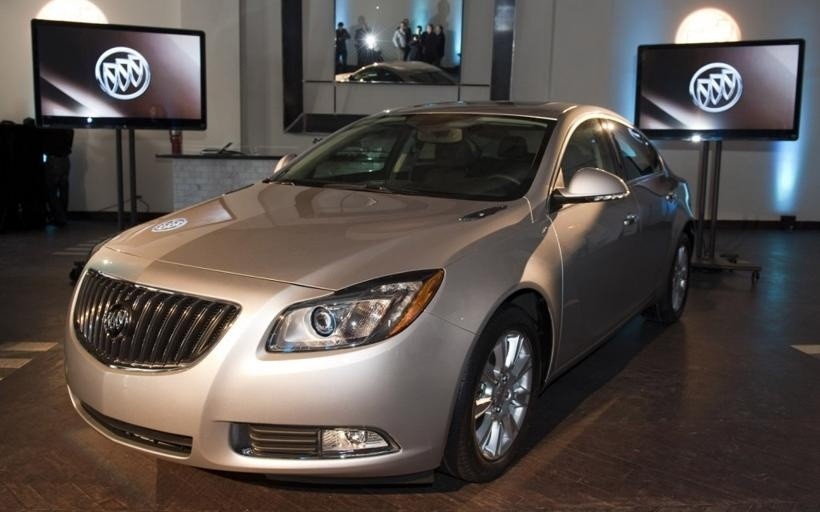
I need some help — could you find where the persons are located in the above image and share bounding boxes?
[354,16,385,66]
[335,21,352,68]
[391,17,446,69]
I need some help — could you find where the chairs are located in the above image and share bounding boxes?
[422,136,533,195]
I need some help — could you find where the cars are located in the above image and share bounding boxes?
[335,61,456,85]
[65,99,698,485]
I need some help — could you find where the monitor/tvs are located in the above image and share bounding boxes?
[31,18,208,131]
[634,38,806,141]
